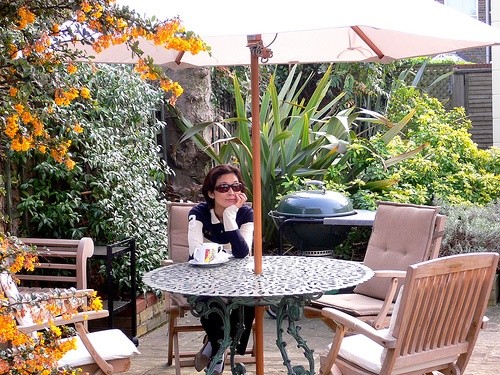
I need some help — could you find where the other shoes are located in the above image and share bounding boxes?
[194,335,213,372]
[212,349,227,375]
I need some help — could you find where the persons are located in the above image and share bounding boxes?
[188,165,254,375]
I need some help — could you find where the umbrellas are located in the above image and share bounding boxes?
[13,0,500,375]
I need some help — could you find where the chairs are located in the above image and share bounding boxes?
[167,203,256,375]
[319,253,500,375]
[303,202,446,375]
[0,271,139,375]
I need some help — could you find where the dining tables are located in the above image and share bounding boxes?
[141,255,374,374]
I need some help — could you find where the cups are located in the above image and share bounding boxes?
[200,243,222,259]
[194,246,211,263]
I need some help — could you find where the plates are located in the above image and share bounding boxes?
[189,259,229,266]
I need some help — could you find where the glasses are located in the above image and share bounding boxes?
[214,183,243,193]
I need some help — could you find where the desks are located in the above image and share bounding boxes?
[26,237,138,349]
